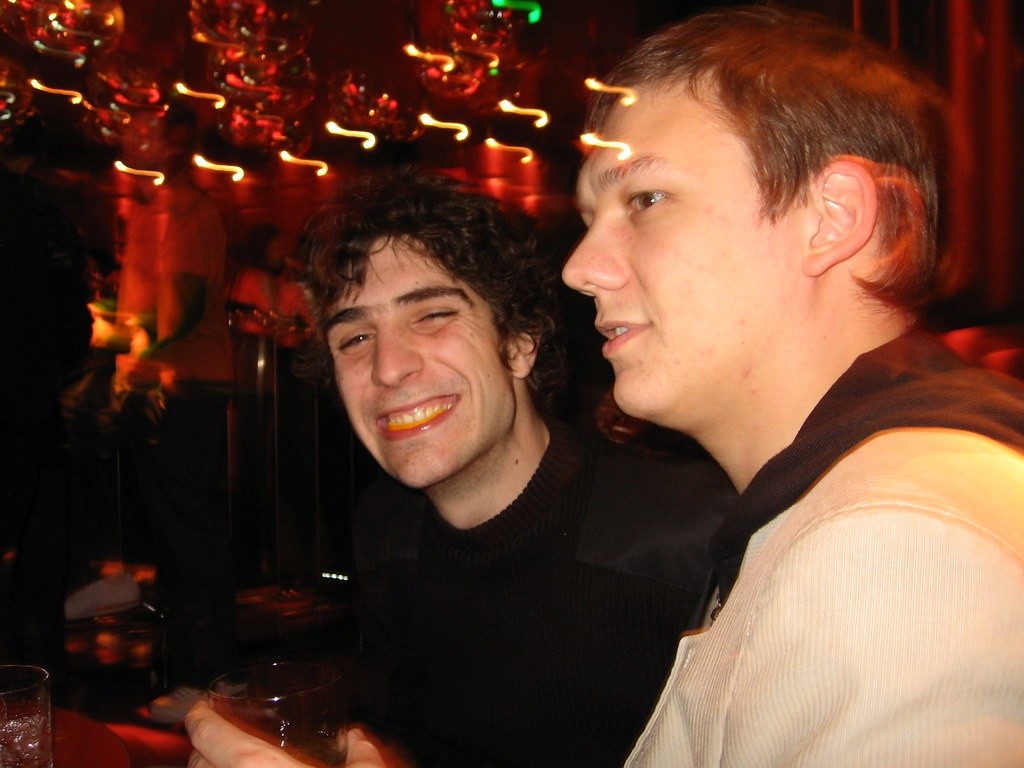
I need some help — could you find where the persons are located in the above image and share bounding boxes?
[0,1,1024,768]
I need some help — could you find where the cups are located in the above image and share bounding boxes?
[0,664,54,768]
[207,661,348,767]
